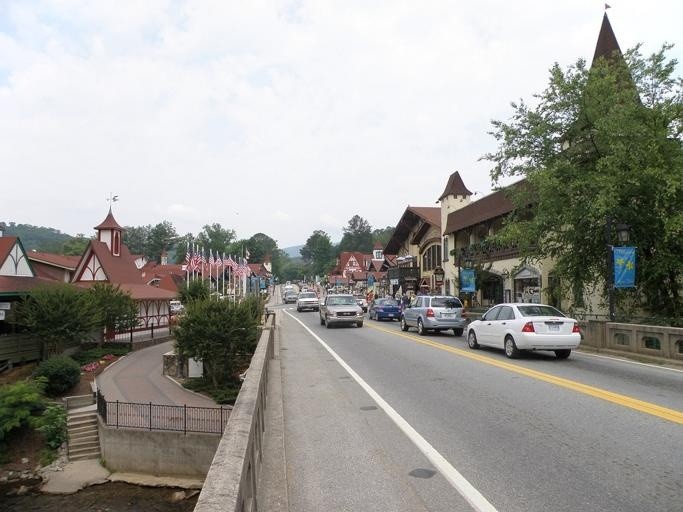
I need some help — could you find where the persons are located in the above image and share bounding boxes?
[375,292,378,300]
[522,289,540,303]
[385,291,416,309]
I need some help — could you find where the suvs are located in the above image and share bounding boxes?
[283,284,319,312]
[401,296,466,336]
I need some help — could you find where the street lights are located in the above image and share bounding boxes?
[605,216,631,322]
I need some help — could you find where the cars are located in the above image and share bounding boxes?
[369,298,402,321]
[467,303,582,359]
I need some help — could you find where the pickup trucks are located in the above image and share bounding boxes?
[320,294,364,328]
[327,286,349,294]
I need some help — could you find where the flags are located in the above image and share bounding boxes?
[185,246,251,277]
